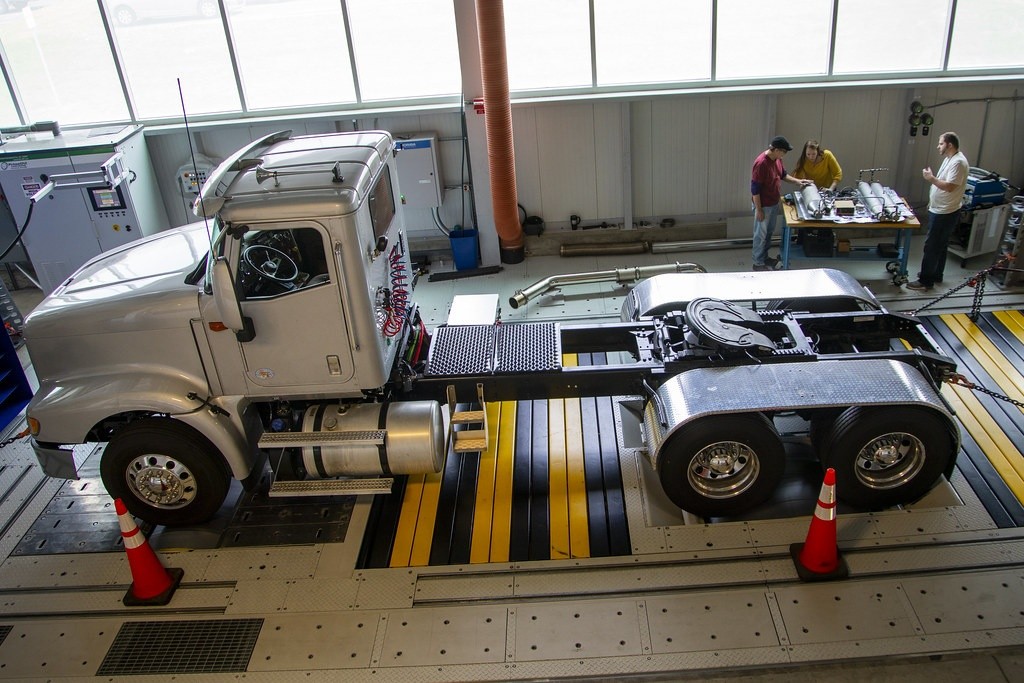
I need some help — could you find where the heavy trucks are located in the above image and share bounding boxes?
[22,125,962,521]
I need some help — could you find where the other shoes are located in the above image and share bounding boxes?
[753,258,776,271]
[908,272,943,290]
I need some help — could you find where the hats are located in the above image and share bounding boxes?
[771,135,794,151]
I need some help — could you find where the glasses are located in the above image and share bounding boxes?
[779,148,787,154]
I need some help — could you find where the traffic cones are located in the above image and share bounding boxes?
[112,497,185,606]
[789,467,855,583]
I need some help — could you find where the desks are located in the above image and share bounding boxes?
[778,190,921,277]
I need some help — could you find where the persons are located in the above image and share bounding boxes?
[750,136,813,272]
[905,132,970,291]
[790,140,843,194]
